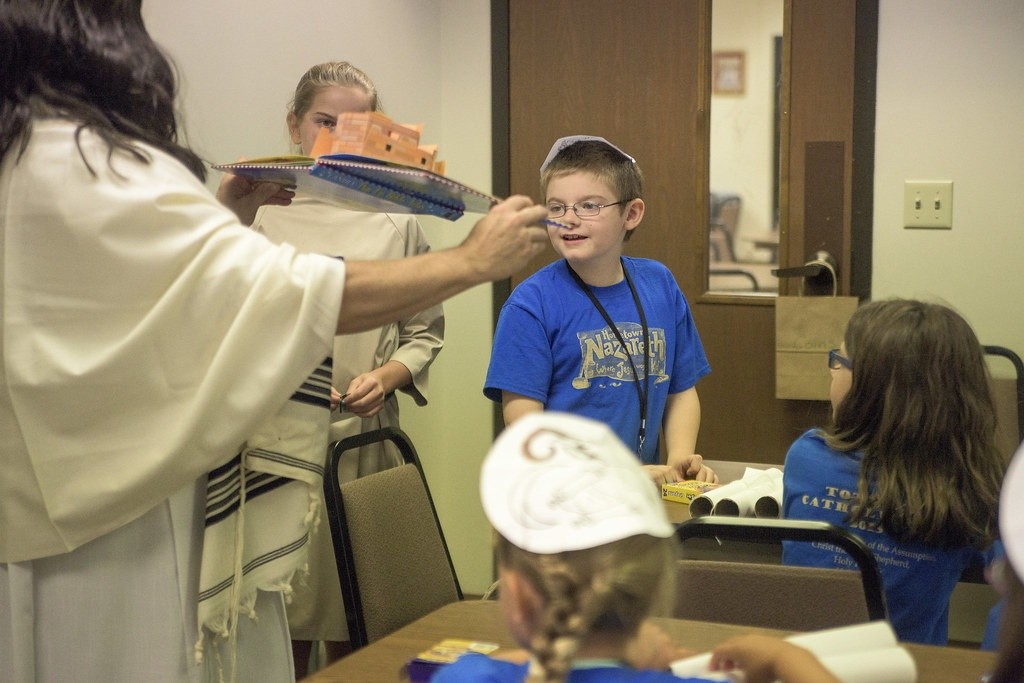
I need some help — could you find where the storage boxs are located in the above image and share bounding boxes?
[661,476,725,506]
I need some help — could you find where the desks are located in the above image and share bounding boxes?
[293,599,1004,683]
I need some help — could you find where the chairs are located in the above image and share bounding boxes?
[709,195,778,293]
[978,342,1024,468]
[326,423,464,651]
[666,515,890,634]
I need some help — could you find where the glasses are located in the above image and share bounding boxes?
[542,199,634,218]
[827,348,854,370]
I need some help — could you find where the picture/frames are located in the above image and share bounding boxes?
[712,52,745,96]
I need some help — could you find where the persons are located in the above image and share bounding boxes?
[1,0,547,683]
[484,134,720,488]
[982,442,1024,683]
[429,411,843,683]
[782,299,1006,646]
[250,62,446,683]
